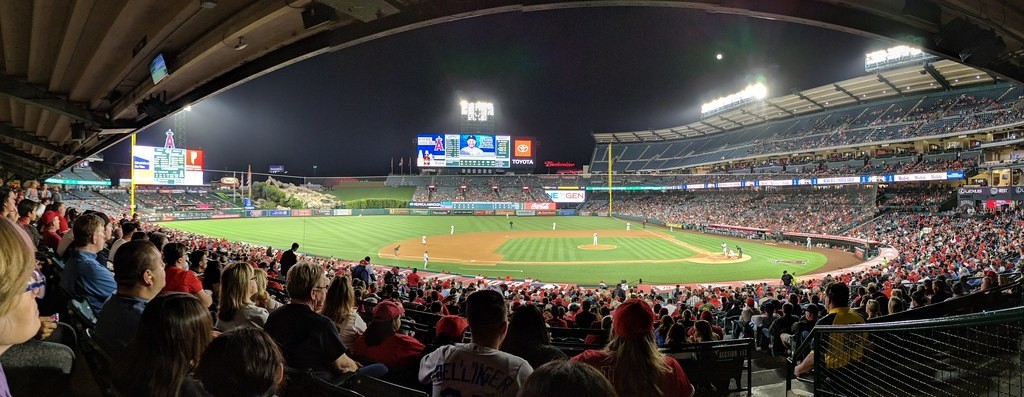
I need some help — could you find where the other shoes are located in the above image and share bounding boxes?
[784,349,792,355]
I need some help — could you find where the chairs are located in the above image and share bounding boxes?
[264,294,759,397]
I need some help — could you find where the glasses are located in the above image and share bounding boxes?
[250,275,258,281]
[316,285,329,290]
[24,269,46,299]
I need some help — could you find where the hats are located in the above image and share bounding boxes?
[360,260,369,265]
[613,300,654,340]
[467,135,476,141]
[436,315,469,337]
[986,271,996,278]
[1005,263,1014,268]
[373,301,405,322]
[42,210,60,224]
[701,311,712,321]
[52,185,58,190]
[659,308,668,314]
[801,303,819,314]
[392,268,398,273]
[747,298,754,305]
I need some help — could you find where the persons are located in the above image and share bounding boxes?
[0,178,390,397]
[721,242,727,258]
[459,135,485,156]
[643,220,645,229]
[413,175,575,202]
[727,247,735,260]
[736,244,743,259]
[510,221,513,229]
[424,250,428,270]
[578,160,747,238]
[745,128,816,246]
[627,221,631,231]
[352,267,725,397]
[451,224,455,234]
[552,222,556,231]
[720,271,1024,397]
[393,245,401,258]
[812,94,1024,273]
[422,234,426,245]
[593,231,598,246]
[806,236,812,251]
[507,213,509,218]
[671,225,673,232]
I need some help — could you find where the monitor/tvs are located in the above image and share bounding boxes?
[148,50,171,85]
